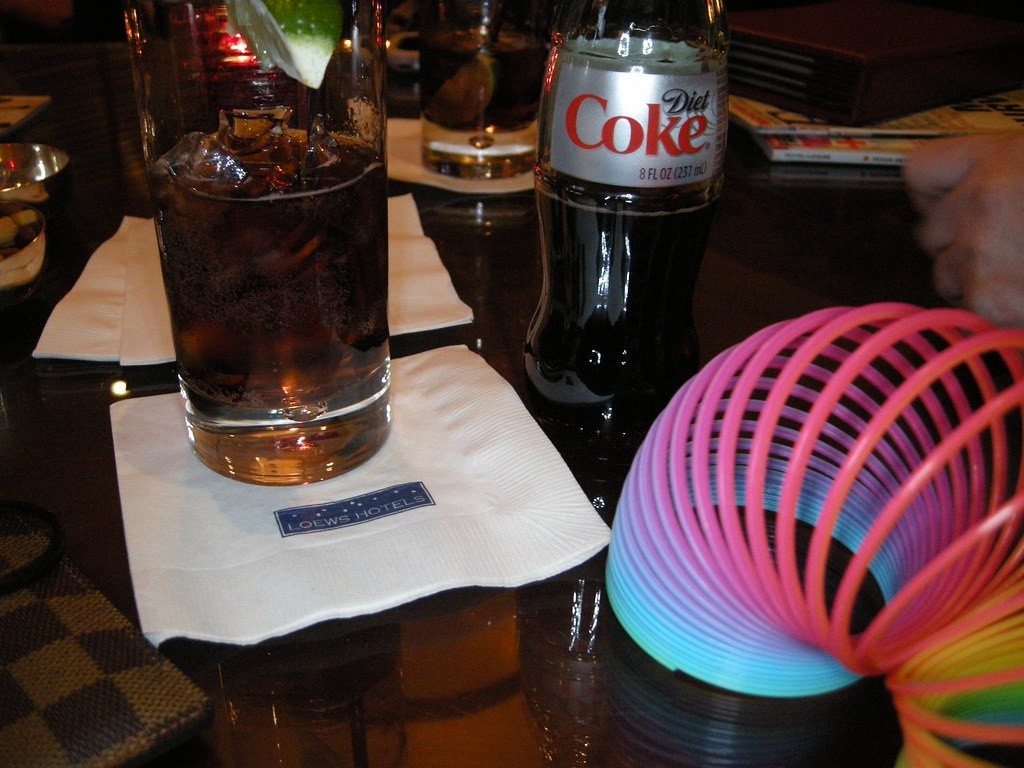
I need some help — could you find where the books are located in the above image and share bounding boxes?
[728,93,1024,168]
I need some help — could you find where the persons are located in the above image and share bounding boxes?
[904,132,1024,327]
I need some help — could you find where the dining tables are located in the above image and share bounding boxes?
[0,99,1024,768]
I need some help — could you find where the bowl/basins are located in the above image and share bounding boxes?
[0,206,72,306]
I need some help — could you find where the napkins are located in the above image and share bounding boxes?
[110,343,611,649]
[384,117,540,195]
[32,193,474,368]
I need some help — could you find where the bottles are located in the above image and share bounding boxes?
[519,0,732,444]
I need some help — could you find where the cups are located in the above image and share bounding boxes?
[421,0,551,179]
[122,1,391,487]
